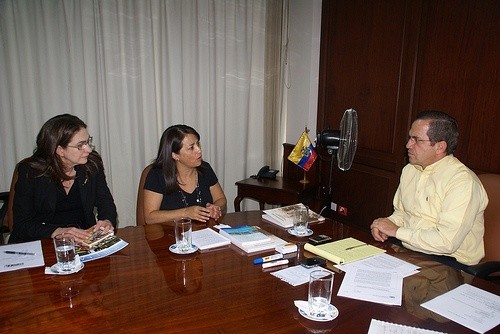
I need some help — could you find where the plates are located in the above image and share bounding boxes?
[287,227,313,237]
[299,302,340,321]
[169,244,198,255]
[51,263,85,275]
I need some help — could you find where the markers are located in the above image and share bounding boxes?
[262,257,294,268]
[253,254,284,264]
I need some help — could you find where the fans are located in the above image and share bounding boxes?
[316,109,358,197]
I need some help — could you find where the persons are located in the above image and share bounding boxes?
[402,253,475,322]
[7,114,119,244]
[142,124,227,223]
[372,109,489,277]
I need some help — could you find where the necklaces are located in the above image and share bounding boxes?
[63,184,70,188]
[178,171,201,207]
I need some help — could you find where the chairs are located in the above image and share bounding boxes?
[463,174,500,277]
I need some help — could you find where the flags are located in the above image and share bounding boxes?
[288,131,317,171]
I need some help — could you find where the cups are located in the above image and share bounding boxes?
[173,217,192,251]
[308,270,334,312]
[54,233,76,271]
[293,205,309,234]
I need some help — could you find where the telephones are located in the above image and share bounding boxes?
[256,166,280,180]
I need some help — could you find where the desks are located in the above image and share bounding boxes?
[234,174,352,216]
[0,210,500,334]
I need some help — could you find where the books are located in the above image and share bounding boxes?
[74,225,129,263]
[220,225,287,253]
[262,203,325,228]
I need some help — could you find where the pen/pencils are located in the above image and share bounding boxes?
[346,243,368,250]
[3,251,35,255]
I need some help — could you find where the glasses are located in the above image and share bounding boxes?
[408,135,441,143]
[59,137,93,150]
